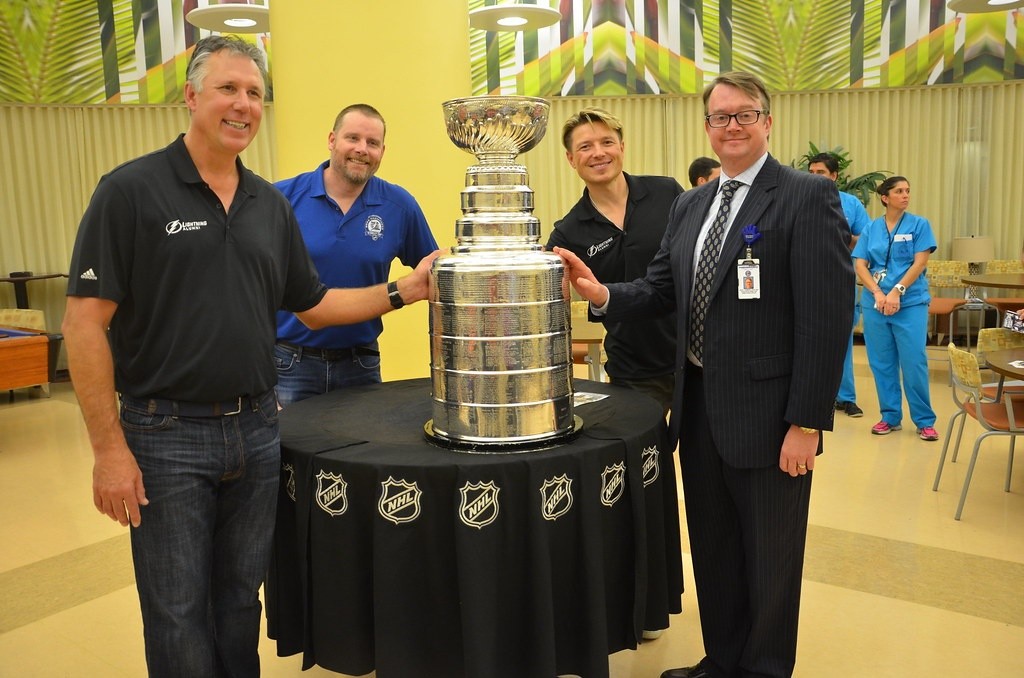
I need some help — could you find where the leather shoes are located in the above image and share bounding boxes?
[659,661,709,678]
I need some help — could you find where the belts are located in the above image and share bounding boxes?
[121,389,274,417]
[274,338,382,360]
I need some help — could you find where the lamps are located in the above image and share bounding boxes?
[952,234,995,304]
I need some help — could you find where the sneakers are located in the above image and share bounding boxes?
[917,427,939,440]
[872,421,902,434]
[834,401,864,417]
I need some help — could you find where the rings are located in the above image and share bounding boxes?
[797,465,806,468]
[893,306,897,308]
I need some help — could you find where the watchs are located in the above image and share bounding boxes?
[894,284,906,295]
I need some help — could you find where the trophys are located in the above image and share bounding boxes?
[424,96,587,456]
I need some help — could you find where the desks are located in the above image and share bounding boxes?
[571,317,605,382]
[986,347,1024,403]
[263,377,684,678]
[0,272,63,389]
[961,273,1024,290]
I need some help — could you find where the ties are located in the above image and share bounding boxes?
[690,179,744,365]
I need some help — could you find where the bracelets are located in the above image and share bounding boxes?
[872,286,880,294]
[387,281,405,309]
[799,426,818,433]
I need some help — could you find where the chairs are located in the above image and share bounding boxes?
[924,259,971,387]
[952,326,1024,463]
[979,259,1024,330]
[570,301,607,381]
[931,342,1024,522]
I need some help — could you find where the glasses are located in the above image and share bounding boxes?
[706,109,765,127]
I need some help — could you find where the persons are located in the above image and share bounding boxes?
[552,70,856,678]
[540,106,685,640]
[851,177,940,440]
[688,157,721,189]
[270,104,440,411]
[1017,308,1024,320]
[808,152,873,419]
[61,32,448,678]
